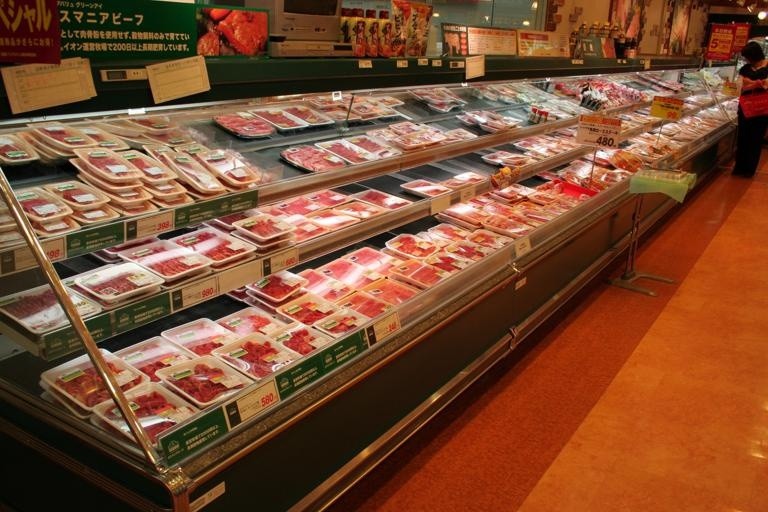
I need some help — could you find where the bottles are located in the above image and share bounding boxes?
[350,8,366,58]
[366,10,379,58]
[569,21,638,61]
[380,9,393,58]
[339,8,352,44]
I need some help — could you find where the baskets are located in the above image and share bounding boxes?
[738,91,768,119]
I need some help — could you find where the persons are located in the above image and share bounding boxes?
[730,40,768,182]
